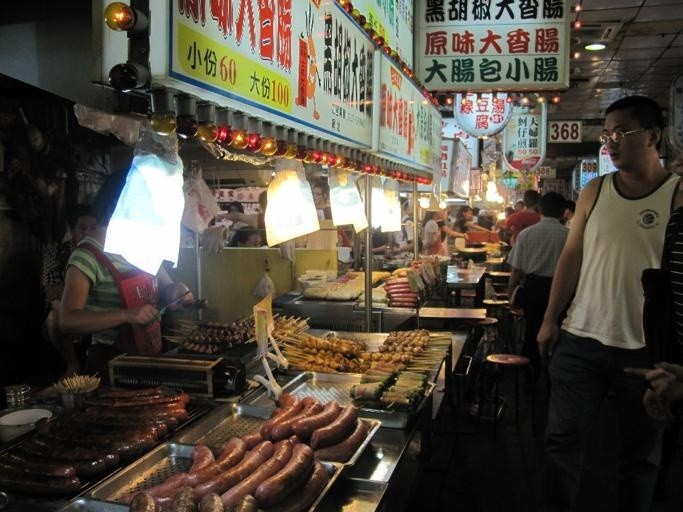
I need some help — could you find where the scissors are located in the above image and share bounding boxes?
[141,289,192,328]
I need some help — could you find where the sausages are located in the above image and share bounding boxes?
[153,441,273,512]
[313,418,368,462]
[0,385,188,471]
[271,394,322,441]
[172,487,198,512]
[198,492,224,512]
[189,440,215,471]
[264,460,328,512]
[0,472,80,496]
[385,278,420,307]
[239,408,284,449]
[235,494,255,512]
[291,401,339,441]
[128,490,155,512]
[255,439,315,509]
[260,394,301,441]
[310,401,357,449]
[220,435,297,509]
[119,436,244,498]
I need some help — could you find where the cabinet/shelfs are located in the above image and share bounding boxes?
[1,326,471,512]
[285,262,487,332]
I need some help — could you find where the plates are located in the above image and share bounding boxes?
[85,442,344,512]
[168,403,382,470]
[271,369,436,416]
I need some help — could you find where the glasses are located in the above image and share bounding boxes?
[599,127,642,143]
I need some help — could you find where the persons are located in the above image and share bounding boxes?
[539,98,682,511]
[227,202,245,228]
[506,193,570,389]
[505,190,541,294]
[60,171,191,376]
[514,201,522,211]
[213,192,265,230]
[447,208,491,246]
[622,164,683,510]
[422,211,442,253]
[564,201,576,227]
[369,198,416,252]
[497,207,512,228]
[228,226,261,248]
[43,206,97,342]
[438,208,468,243]
[311,183,333,219]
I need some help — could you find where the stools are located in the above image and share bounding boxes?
[483,298,509,320]
[482,351,535,432]
[510,308,524,339]
[479,317,498,354]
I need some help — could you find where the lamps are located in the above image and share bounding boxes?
[313,140,324,163]
[197,103,217,144]
[323,142,332,165]
[105,2,149,35]
[350,149,359,170]
[109,60,150,93]
[336,145,345,169]
[303,138,317,162]
[149,90,177,137]
[174,97,198,139]
[231,115,250,150]
[275,129,289,157]
[215,109,234,146]
[260,123,277,157]
[297,134,308,160]
[286,131,298,158]
[357,151,435,186]
[330,144,339,166]
[344,146,352,169]
[246,118,264,152]
